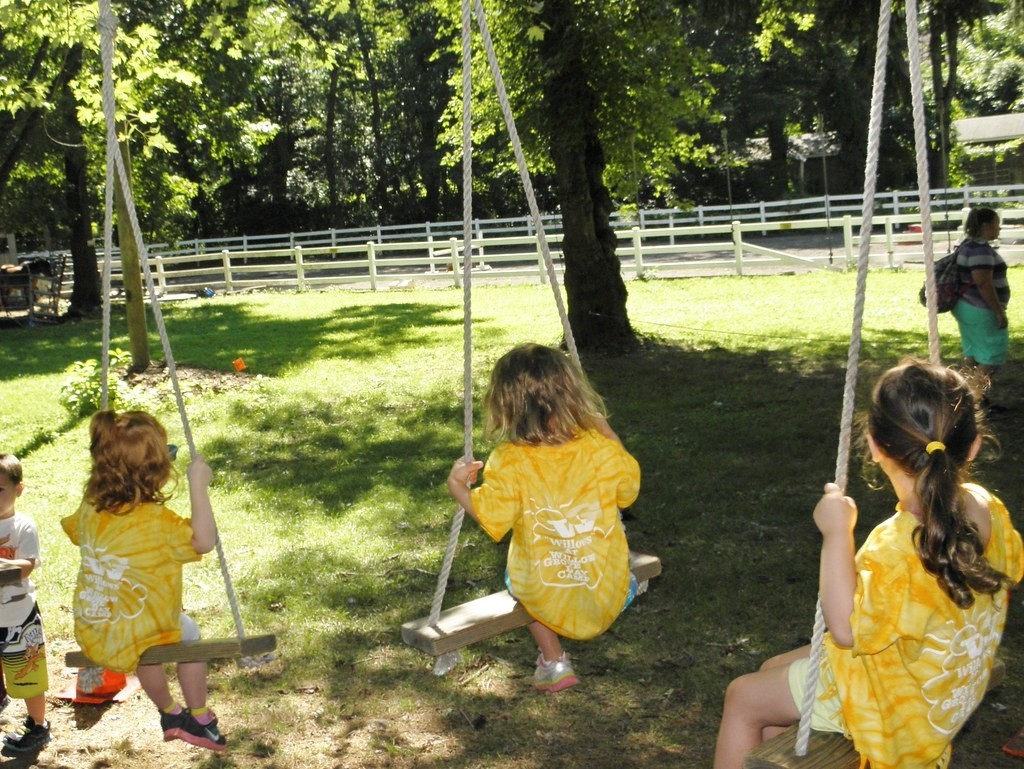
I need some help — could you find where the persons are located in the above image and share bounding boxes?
[61,411,226,749]
[0,454,52,750]
[951,207,1011,407]
[448,342,641,693]
[713,365,1024,769]
[141,577,147,579]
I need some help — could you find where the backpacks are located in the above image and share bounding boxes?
[920,252,965,313]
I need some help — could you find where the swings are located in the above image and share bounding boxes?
[396,0,663,656]
[744,0,948,769]
[64,1,279,669]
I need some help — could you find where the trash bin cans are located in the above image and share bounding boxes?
[0,259,51,312]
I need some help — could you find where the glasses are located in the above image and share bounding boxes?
[168,444,178,462]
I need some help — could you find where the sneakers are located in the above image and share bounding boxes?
[5,723,50,755]
[534,653,579,690]
[157,709,185,739]
[179,706,226,751]
